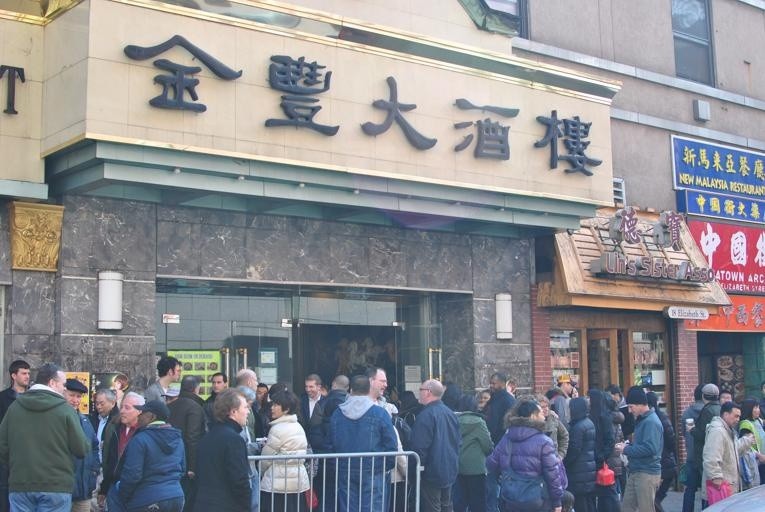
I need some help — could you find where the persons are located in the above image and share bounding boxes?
[252,383,272,420]
[681,383,705,512]
[64,379,100,511]
[326,375,399,512]
[478,372,518,446]
[571,386,579,399]
[139,356,180,405]
[738,427,761,491]
[233,370,266,511]
[0,360,31,424]
[603,392,626,502]
[605,384,635,440]
[701,402,757,511]
[167,374,207,480]
[485,401,568,511]
[586,388,622,511]
[533,394,569,461]
[544,373,577,423]
[718,389,732,407]
[300,374,325,446]
[0,363,91,511]
[563,396,597,511]
[255,383,319,510]
[759,381,765,430]
[452,393,495,512]
[477,390,492,410]
[646,392,680,512]
[117,392,145,462]
[103,400,186,512]
[561,491,575,512]
[204,373,227,402]
[113,375,130,392]
[257,391,311,511]
[193,388,251,512]
[91,389,122,511]
[364,366,408,511]
[309,374,350,511]
[405,378,463,512]
[615,386,664,511]
[738,399,765,465]
[685,383,721,511]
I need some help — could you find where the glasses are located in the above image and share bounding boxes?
[419,388,431,392]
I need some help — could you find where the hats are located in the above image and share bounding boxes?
[557,374,577,386]
[701,383,719,395]
[626,386,648,404]
[64,379,88,393]
[133,400,171,419]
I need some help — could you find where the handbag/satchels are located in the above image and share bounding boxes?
[500,439,547,511]
[706,479,731,506]
[305,487,318,510]
